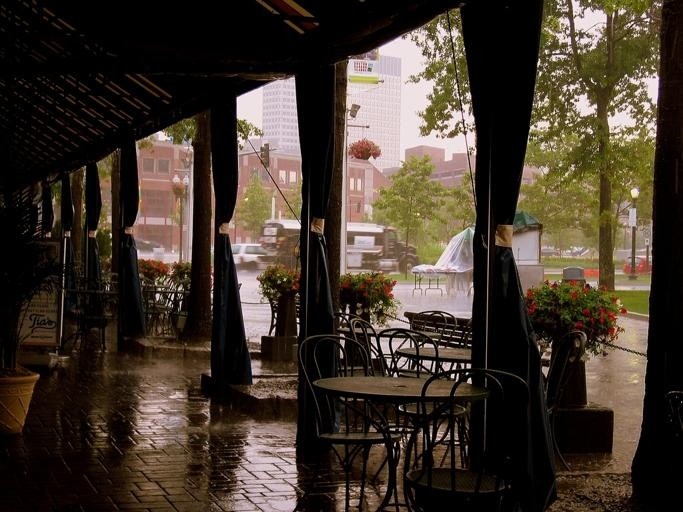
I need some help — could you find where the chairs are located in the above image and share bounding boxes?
[299,302,554,512]
[91,260,206,337]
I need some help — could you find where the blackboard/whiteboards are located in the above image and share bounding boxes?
[17,236,67,346]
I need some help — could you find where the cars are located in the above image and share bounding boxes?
[541,248,559,256]
[623,256,652,274]
[231,243,276,271]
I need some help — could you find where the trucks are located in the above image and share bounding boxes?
[258,220,419,274]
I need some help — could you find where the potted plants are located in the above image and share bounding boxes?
[1,201,53,438]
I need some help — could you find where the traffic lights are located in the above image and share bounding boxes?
[260,143,270,167]
[357,203,361,213]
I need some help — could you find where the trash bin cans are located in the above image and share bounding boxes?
[562,266,586,291]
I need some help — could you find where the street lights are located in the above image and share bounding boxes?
[628,188,640,279]
[172,169,192,260]
